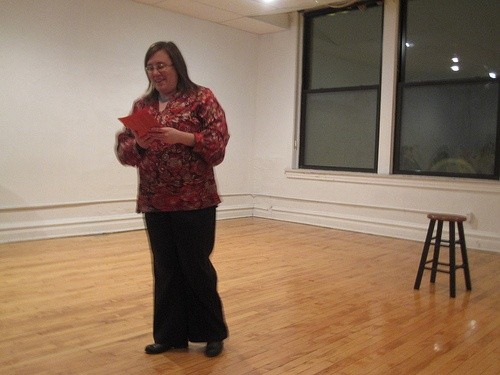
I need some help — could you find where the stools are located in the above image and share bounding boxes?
[414,212,472,298]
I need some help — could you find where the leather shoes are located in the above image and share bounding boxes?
[145,344,188,354]
[206,341,223,357]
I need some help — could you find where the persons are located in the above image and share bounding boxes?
[426,140,495,176]
[114,42,230,358]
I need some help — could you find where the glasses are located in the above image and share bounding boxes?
[146,63,174,72]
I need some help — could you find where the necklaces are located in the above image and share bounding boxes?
[158,97,170,103]
